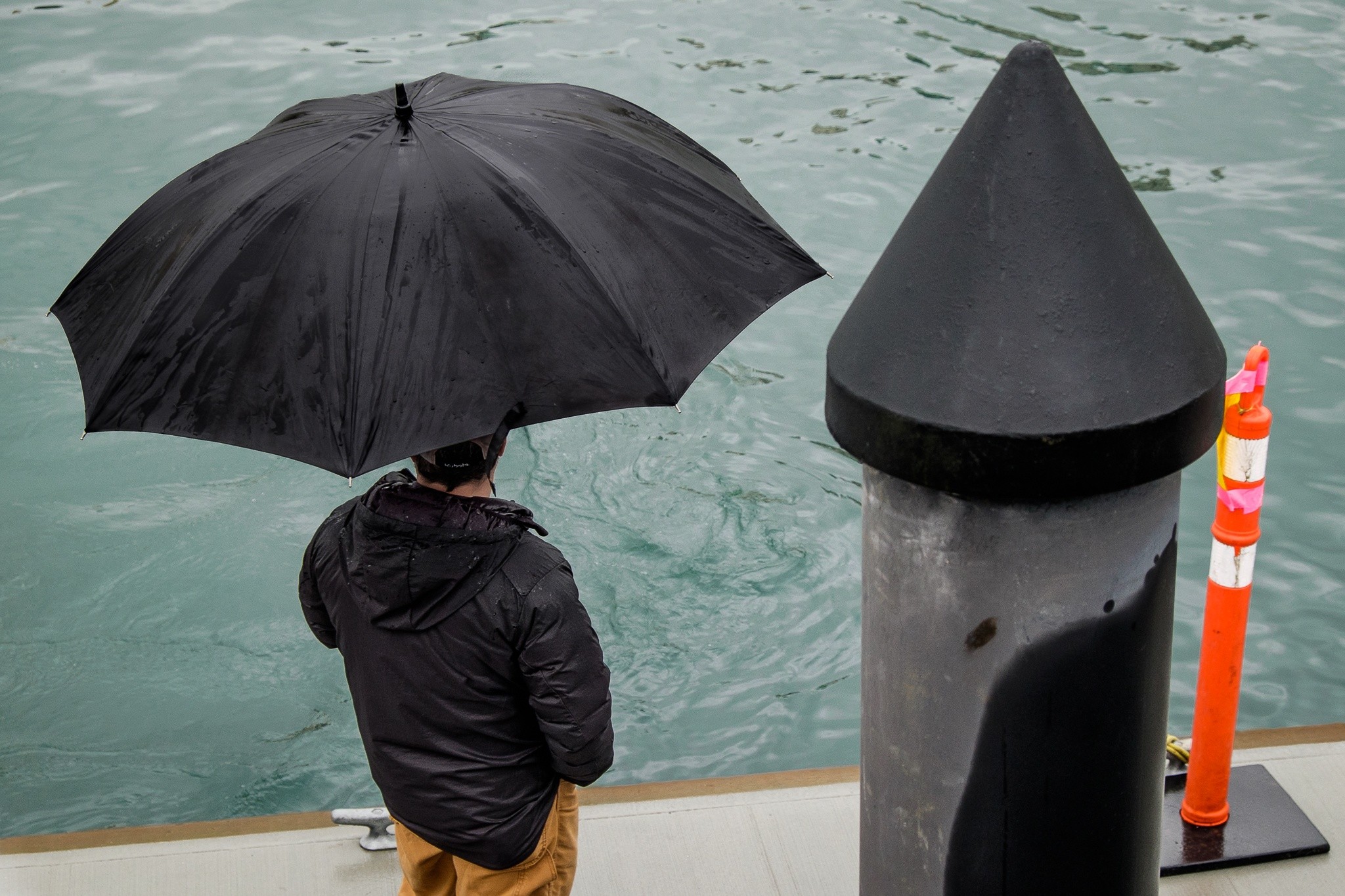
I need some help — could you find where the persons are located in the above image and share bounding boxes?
[298,432,615,895]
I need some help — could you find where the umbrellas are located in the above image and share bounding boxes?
[44,72,837,489]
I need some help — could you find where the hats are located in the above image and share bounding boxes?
[418,430,501,468]
